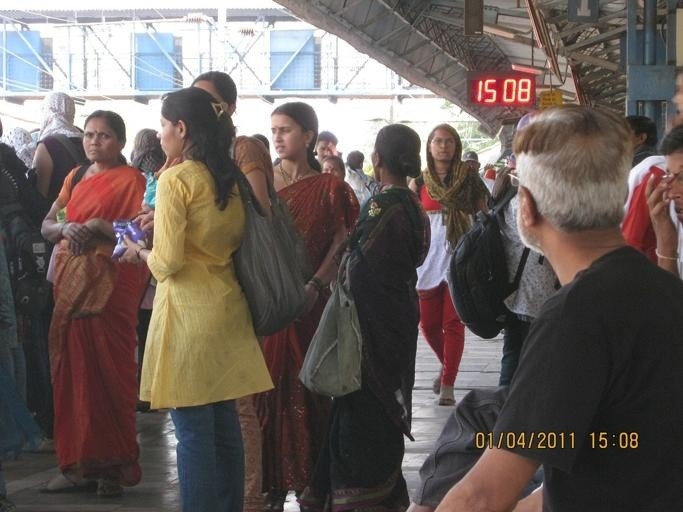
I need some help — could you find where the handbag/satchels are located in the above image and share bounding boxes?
[235,171,361,397]
[46,242,58,285]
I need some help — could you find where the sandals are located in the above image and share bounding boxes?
[97,480,123,496]
[39,471,98,492]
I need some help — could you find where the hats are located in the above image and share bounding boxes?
[463,152,480,168]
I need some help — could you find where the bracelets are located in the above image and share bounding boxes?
[136,246,147,257]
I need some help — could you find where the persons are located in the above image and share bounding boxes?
[39,109,152,499]
[1,93,89,510]
[616,65,682,278]
[492,149,560,388]
[407,123,492,407]
[119,88,274,511]
[405,107,682,512]
[464,149,481,176]
[129,129,166,410]
[251,101,359,511]
[295,122,432,512]
[155,70,276,511]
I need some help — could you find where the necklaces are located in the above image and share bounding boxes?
[278,162,312,185]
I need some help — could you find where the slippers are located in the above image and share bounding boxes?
[433,376,456,405]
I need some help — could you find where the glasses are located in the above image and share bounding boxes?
[508,170,525,187]
[430,139,455,145]
[663,173,683,183]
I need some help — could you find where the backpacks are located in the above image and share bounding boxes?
[446,185,529,339]
[3,203,51,317]
